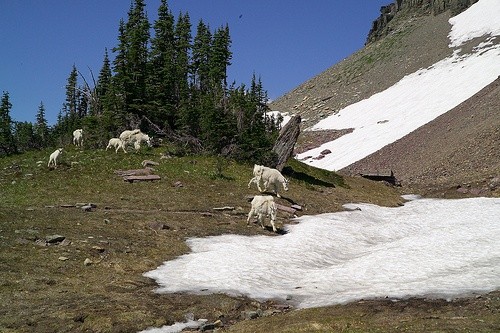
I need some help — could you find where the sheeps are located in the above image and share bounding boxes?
[247,195,278,232]
[48,149,62,167]
[106,129,151,154]
[248,164,289,198]
[73,129,84,147]
